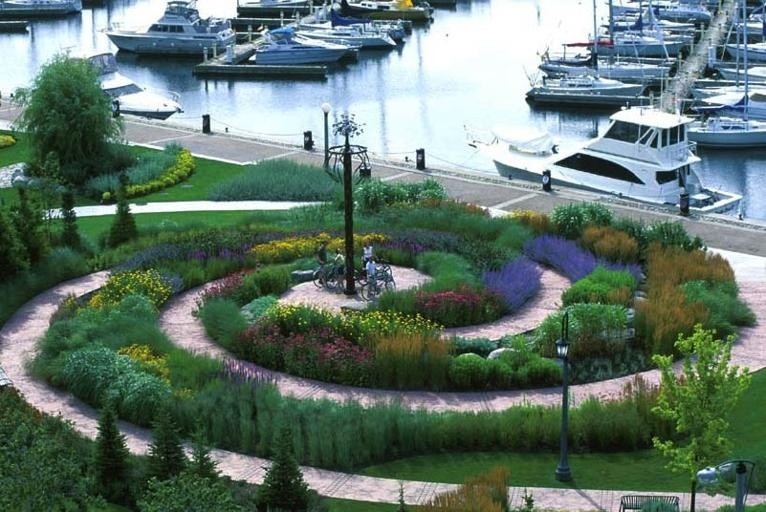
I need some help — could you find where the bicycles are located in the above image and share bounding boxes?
[308,259,397,301]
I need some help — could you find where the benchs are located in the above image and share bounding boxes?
[618,494,681,512]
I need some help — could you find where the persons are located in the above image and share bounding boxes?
[365,256,383,299]
[362,241,374,277]
[334,248,346,295]
[317,241,329,285]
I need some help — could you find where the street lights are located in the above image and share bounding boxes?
[322,142,372,297]
[552,310,573,482]
[319,100,331,168]
[696,457,757,512]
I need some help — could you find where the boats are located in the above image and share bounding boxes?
[104,0,239,58]
[65,48,186,123]
[250,0,413,65]
[522,1,766,149]
[0,20,30,32]
[0,0,84,14]
[492,99,745,214]
